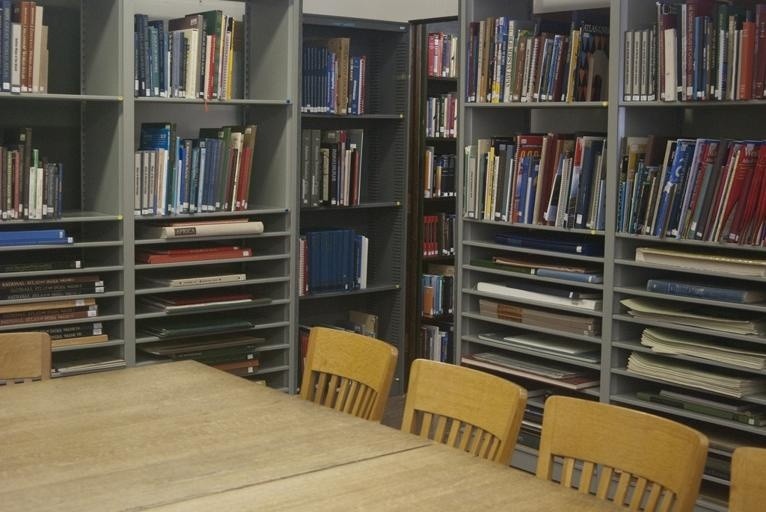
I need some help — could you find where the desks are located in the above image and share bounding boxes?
[0,358,643,512]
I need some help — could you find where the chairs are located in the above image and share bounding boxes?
[0,332,52,386]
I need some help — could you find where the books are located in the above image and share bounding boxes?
[134,122,257,215]
[302,39,367,116]
[133,11,237,102]
[420,31,459,365]
[1,231,127,373]
[2,0,50,94]
[462,20,609,446]
[133,219,267,386]
[297,229,377,385]
[620,3,766,482]
[1,127,62,221]
[301,129,364,205]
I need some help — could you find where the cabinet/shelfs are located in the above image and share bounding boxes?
[457,1,766,511]
[299,12,461,401]
[0,1,303,397]
